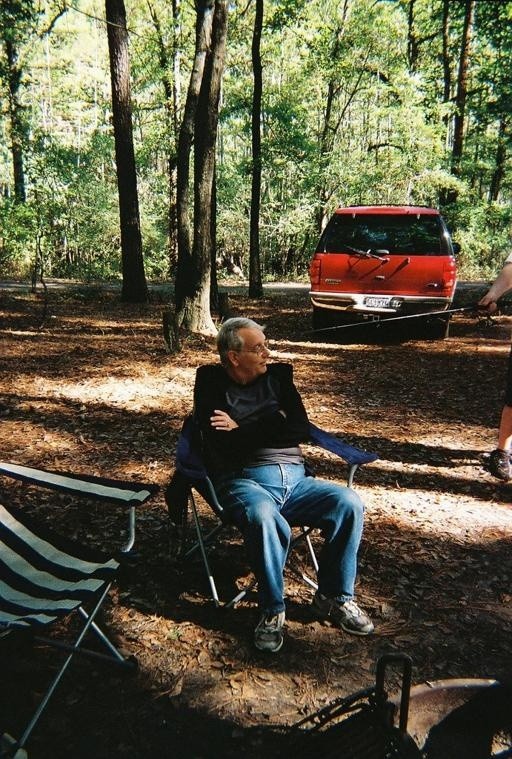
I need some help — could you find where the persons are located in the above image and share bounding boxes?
[477,244,512,483]
[188,313,376,651]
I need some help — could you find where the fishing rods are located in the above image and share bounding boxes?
[306,300,510,333]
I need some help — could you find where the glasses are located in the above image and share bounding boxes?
[243,338,269,357]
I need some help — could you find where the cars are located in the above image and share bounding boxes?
[306,204,460,338]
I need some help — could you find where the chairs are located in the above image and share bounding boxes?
[2,460,172,758]
[176,412,378,613]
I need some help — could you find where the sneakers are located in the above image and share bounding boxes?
[312,590,374,635]
[255,610,285,652]
[493,449,512,478]
